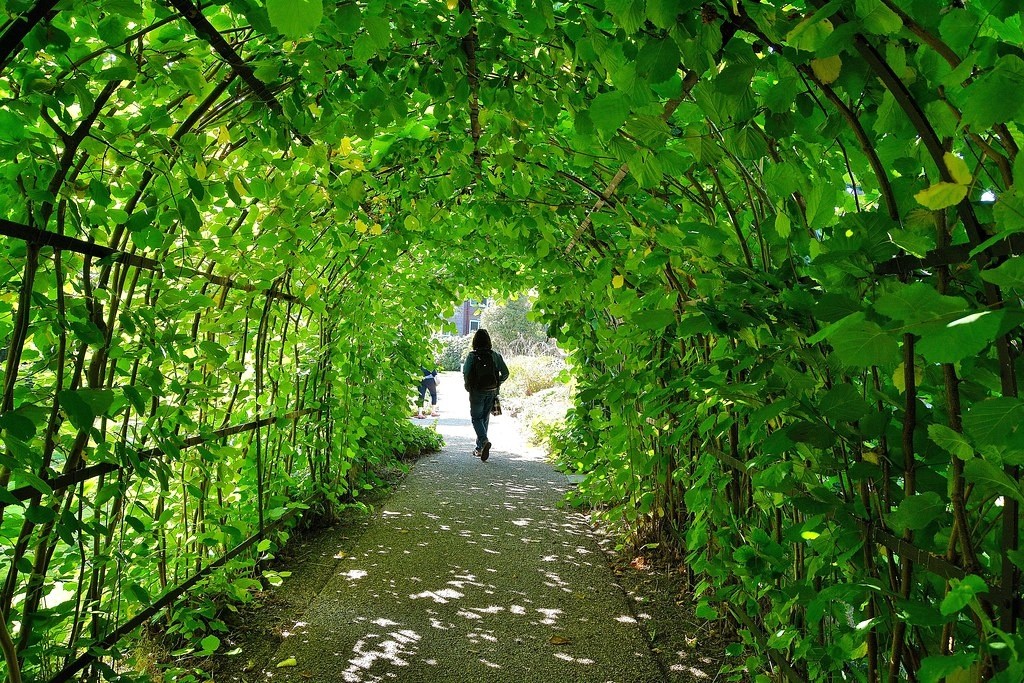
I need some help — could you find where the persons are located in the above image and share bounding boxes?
[463,328,510,462]
[416,365,441,419]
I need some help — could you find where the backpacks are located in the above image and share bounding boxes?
[470,350,496,388]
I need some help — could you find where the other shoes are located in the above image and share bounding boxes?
[481,442,491,461]
[418,415,425,418]
[473,449,482,458]
[431,412,439,416]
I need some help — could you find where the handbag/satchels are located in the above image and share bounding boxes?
[491,397,502,416]
[435,375,440,386]
